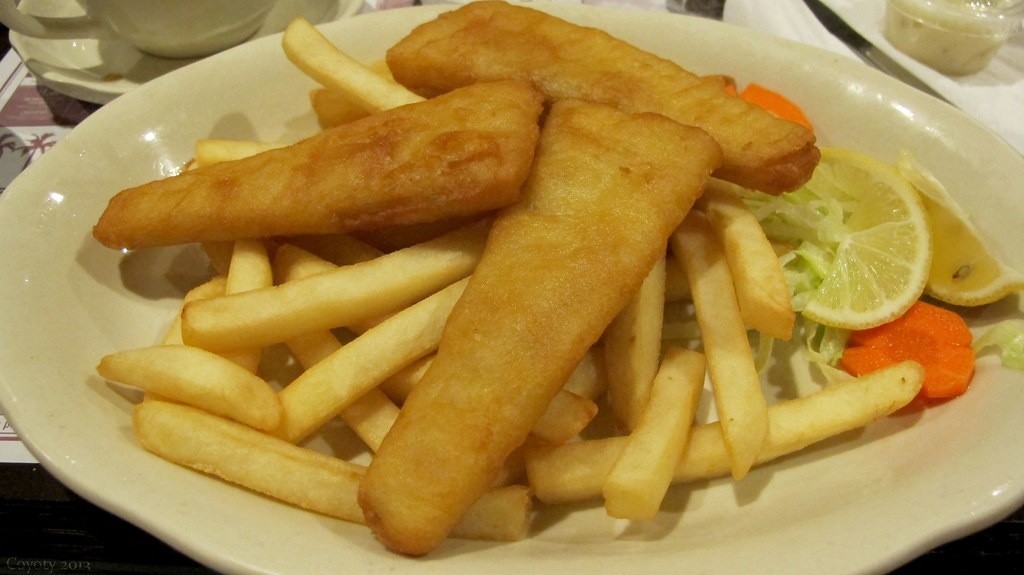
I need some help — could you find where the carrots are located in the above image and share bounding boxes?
[720,75,812,133]
[839,299,977,399]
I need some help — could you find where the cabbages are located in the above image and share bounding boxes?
[732,184,1024,384]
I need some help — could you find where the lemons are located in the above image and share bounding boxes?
[893,151,1024,308]
[801,164,931,330]
[802,145,889,218]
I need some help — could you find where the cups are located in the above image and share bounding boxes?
[882,0,1024,76]
[0,0,276,60]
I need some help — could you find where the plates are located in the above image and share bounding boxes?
[0,0,1024,575]
[6,0,364,106]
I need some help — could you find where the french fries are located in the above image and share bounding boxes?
[90,14,924,540]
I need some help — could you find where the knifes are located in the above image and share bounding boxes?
[803,0,956,110]
